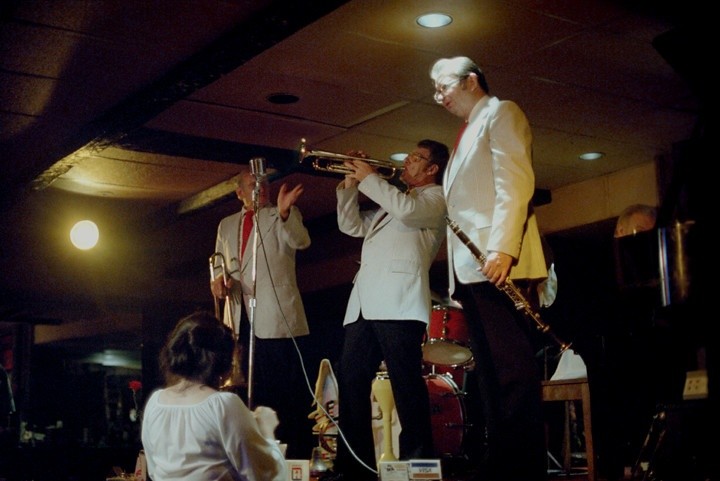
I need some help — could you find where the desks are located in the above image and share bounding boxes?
[540,378,596,481]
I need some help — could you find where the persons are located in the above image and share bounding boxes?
[211,164,313,459]
[430,55,549,481]
[141,310,286,481]
[319,139,450,481]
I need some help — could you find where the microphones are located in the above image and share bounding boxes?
[249,156,268,202]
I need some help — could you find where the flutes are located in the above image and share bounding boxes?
[442,213,574,359]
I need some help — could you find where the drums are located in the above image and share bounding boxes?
[418,304,474,368]
[391,372,468,470]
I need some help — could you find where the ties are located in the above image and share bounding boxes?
[454,123,467,153]
[241,211,255,257]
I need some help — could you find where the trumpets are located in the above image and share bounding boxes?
[297,136,406,180]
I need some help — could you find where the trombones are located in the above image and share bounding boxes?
[207,251,246,389]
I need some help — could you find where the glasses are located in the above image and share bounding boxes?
[434,77,466,100]
[408,152,431,163]
